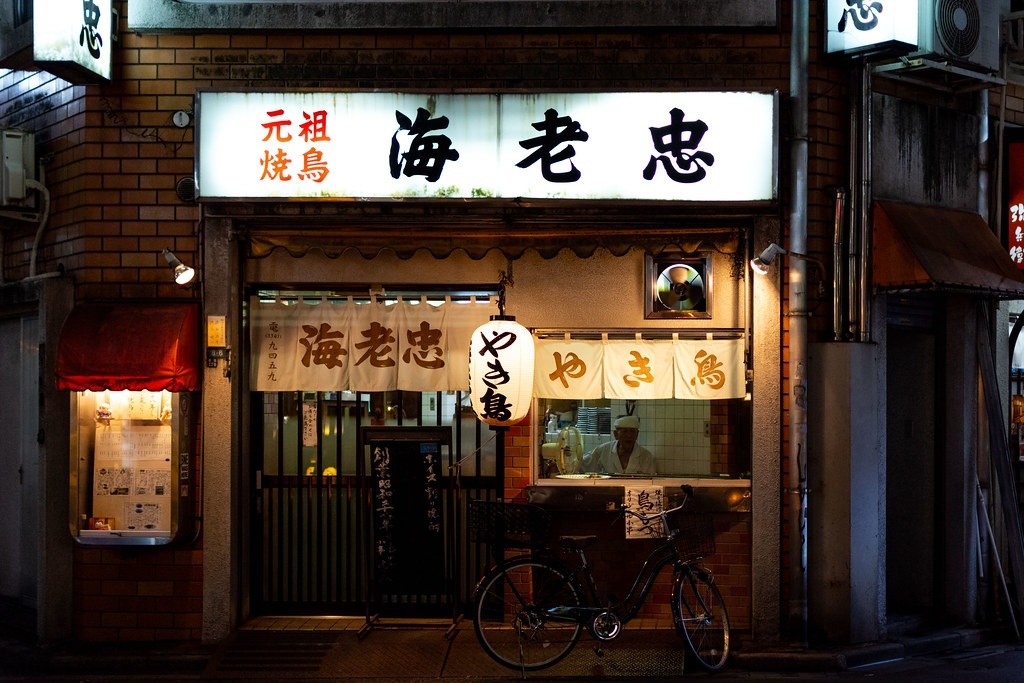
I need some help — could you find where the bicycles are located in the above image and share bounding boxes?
[463,483,733,683]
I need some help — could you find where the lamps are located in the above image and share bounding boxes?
[751,243,790,274]
[161,247,195,284]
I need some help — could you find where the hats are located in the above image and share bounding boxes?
[614,416,640,428]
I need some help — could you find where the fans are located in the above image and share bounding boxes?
[542,425,584,475]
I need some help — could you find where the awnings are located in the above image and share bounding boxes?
[55,300,200,392]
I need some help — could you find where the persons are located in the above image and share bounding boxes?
[574,416,653,474]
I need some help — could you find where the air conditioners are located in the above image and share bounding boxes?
[896,0,1002,81]
[0,129,36,209]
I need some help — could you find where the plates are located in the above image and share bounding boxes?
[556,475,612,479]
[577,407,611,434]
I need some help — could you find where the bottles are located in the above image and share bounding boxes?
[548,415,558,433]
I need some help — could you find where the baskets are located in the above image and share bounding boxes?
[466,500,550,550]
[649,512,716,567]
